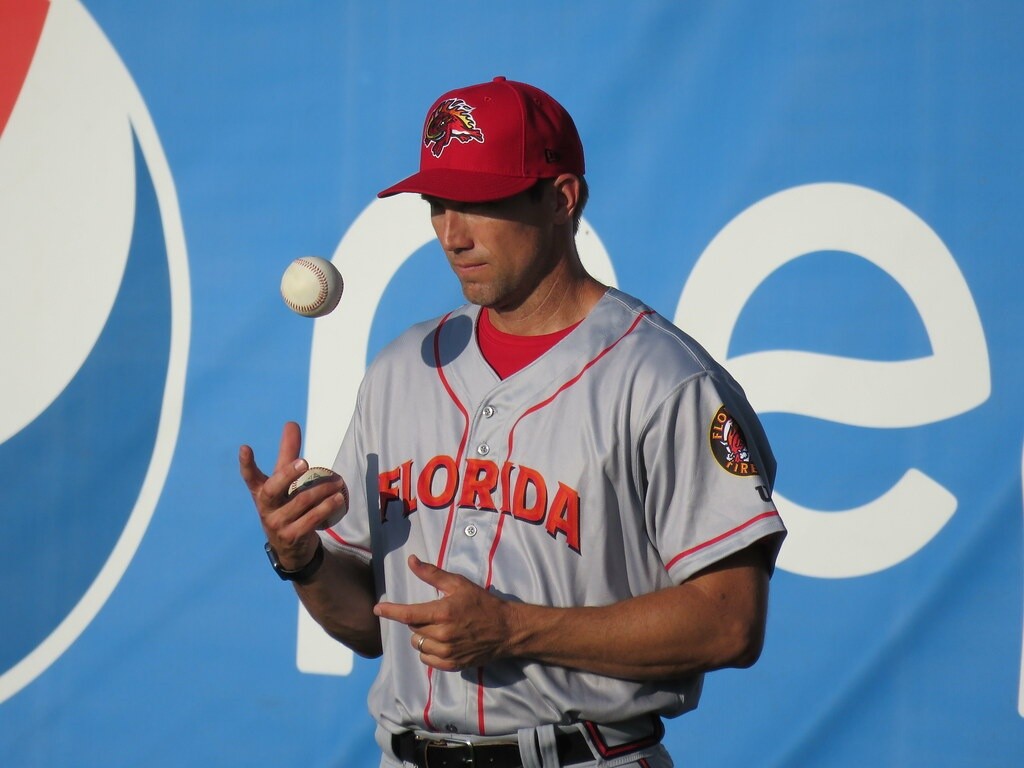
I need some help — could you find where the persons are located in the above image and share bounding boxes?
[239,76,790,768]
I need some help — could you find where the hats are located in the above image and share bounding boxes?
[378,74,586,202]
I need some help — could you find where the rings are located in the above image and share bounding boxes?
[418,637,427,654]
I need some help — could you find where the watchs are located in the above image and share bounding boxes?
[264,537,324,583]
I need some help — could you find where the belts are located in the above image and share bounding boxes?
[391,728,595,768]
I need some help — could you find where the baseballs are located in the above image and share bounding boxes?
[279,255,344,320]
[288,466,351,531]
